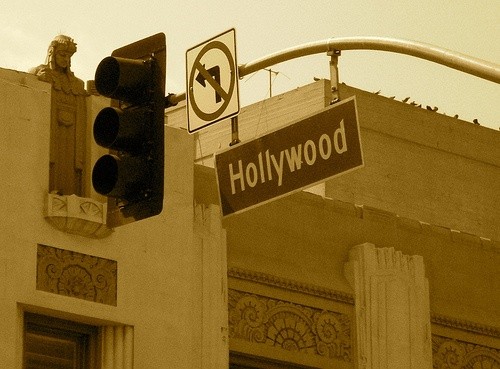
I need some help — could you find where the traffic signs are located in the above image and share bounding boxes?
[186,27,239,133]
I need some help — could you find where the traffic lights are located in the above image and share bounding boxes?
[93,32,166,228]
